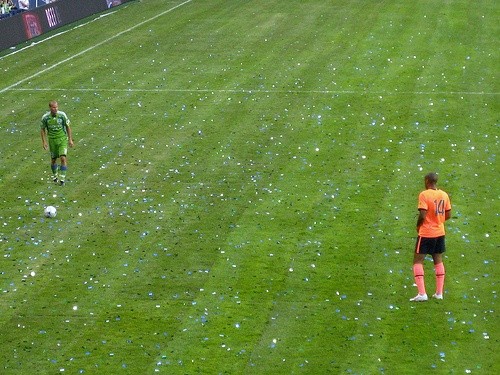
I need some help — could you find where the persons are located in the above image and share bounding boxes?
[40,101,73,186]
[409,173,451,302]
[0,0,29,13]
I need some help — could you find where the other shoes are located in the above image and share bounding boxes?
[60,178,66,183]
[410,294,428,302]
[431,292,444,300]
[53,177,59,182]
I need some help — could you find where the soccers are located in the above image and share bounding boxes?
[44,206,57,218]
